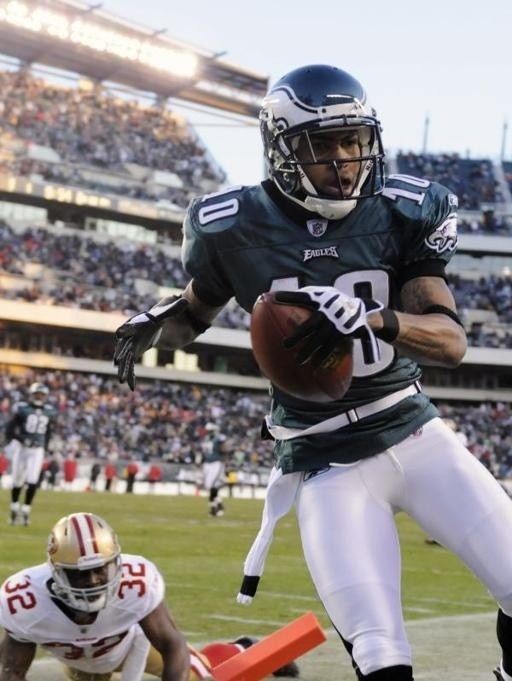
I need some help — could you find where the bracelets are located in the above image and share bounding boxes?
[372,307,399,345]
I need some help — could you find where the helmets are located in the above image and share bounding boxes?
[49,511,123,595]
[30,383,50,397]
[260,63,381,178]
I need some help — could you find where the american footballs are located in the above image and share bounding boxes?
[251,290,353,403]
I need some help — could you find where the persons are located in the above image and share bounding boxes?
[1,510,303,681]
[1,221,512,500]
[195,423,231,520]
[1,73,228,211]
[395,151,512,219]
[107,62,512,681]
[1,382,60,529]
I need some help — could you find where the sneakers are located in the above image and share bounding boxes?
[234,634,300,678]
[8,515,32,528]
[207,504,224,517]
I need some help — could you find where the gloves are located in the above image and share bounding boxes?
[112,294,189,393]
[272,285,386,370]
